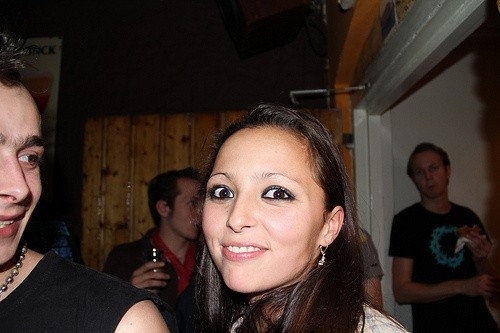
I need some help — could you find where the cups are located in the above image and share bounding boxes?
[144,248,167,281]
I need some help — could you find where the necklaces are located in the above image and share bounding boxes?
[0,243,28,296]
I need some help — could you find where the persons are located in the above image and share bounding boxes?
[0,35,178,333]
[103,168,202,332]
[388,142,500,333]
[192,105,410,333]
